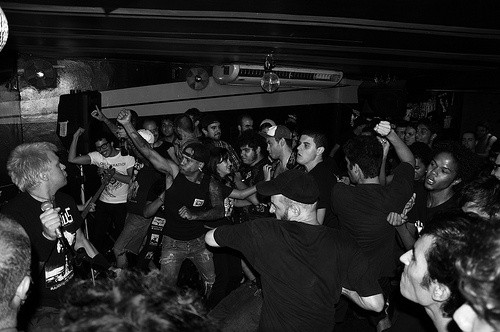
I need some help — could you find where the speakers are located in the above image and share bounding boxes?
[56,89,102,156]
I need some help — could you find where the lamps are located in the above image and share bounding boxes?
[261,53,280,92]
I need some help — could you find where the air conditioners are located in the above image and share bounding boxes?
[212,64,343,87]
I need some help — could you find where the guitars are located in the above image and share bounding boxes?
[68,169,115,236]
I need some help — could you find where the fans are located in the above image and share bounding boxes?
[23,56,56,88]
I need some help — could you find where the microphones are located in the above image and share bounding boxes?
[41,201,69,251]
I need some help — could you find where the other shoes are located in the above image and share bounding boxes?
[375,317,392,332]
[353,311,366,320]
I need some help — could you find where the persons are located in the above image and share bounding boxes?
[0,106,500,332]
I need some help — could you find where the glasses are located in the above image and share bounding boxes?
[493,164,500,170]
[96,143,109,152]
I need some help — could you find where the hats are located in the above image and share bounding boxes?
[257,169,318,204]
[260,119,276,127]
[182,143,210,170]
[267,125,292,139]
[136,129,154,144]
[198,114,219,130]
